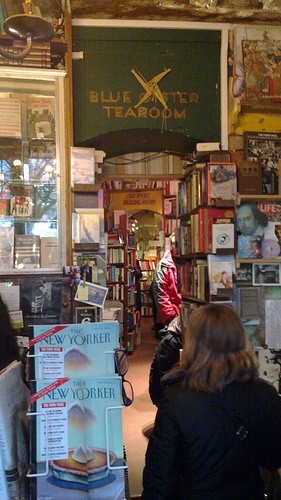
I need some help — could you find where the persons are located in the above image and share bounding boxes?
[237,205,266,238]
[148,315,183,410]
[141,303,281,500]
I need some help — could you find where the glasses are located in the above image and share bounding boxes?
[115,350,134,407]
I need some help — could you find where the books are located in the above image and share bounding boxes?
[34,323,121,381]
[70,142,237,353]
[0,93,59,334]
[37,376,124,500]
[0,360,29,499]
